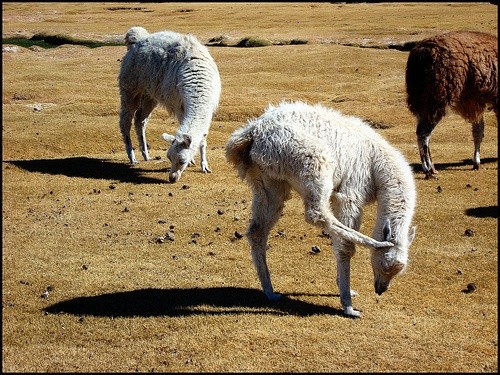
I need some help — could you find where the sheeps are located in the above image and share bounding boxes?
[405,31,498,179]
[118,26,221,184]
[223,101,418,319]
